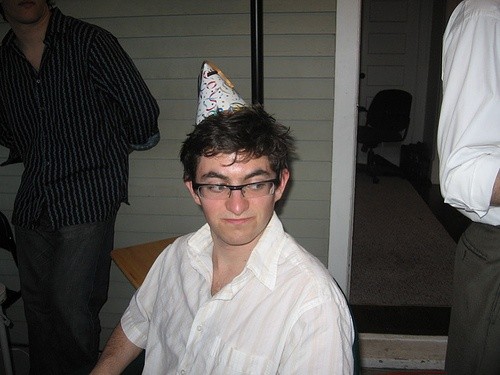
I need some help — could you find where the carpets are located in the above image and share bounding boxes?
[349,176,458,307]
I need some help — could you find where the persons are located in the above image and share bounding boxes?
[90,106,356,375]
[437,0,499,374]
[0,0,161,375]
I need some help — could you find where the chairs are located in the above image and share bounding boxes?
[111,237,181,287]
[358,89,414,182]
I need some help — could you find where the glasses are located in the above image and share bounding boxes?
[194,173,282,199]
